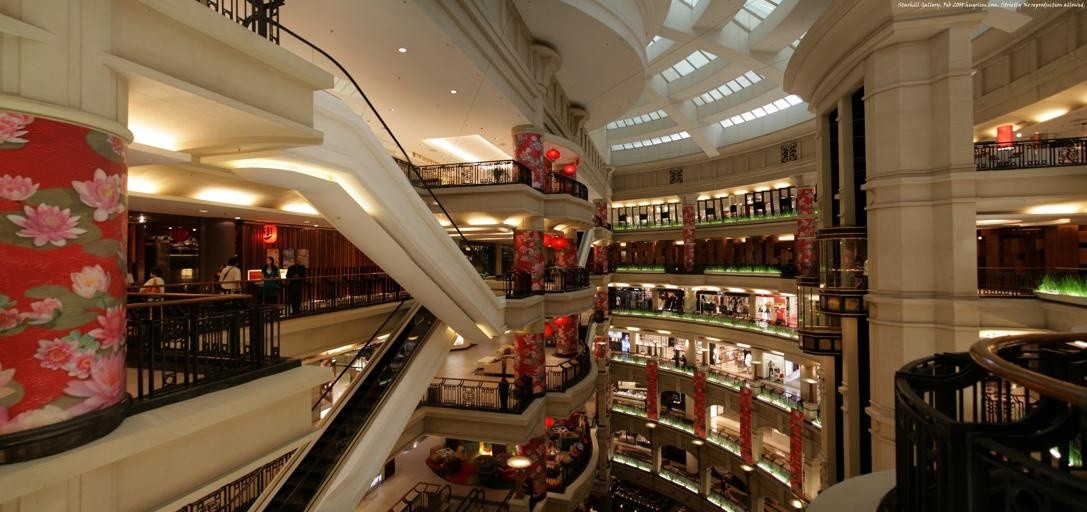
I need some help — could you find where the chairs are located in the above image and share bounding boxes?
[497,345,515,360]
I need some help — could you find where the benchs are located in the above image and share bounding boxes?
[477,356,497,369]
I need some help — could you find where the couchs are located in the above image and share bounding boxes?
[483,358,514,376]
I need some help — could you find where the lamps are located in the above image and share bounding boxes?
[545,416,555,429]
[563,162,577,175]
[543,234,552,247]
[263,223,277,244]
[544,324,554,337]
[550,237,568,252]
[507,454,532,469]
[553,317,571,325]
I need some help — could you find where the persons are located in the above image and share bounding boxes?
[854,257,868,289]
[720,479,726,496]
[615,294,620,311]
[218,256,242,294]
[456,440,468,461]
[672,354,679,368]
[287,256,305,314]
[744,351,751,374]
[621,334,630,351]
[138,266,165,300]
[214,263,225,290]
[262,256,281,319]
[320,357,331,367]
[767,359,775,378]
[666,395,673,412]
[681,354,687,371]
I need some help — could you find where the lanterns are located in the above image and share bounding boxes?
[262,223,277,245]
[544,233,568,249]
[552,317,568,325]
[545,323,552,337]
[545,417,554,426]
[564,164,576,175]
